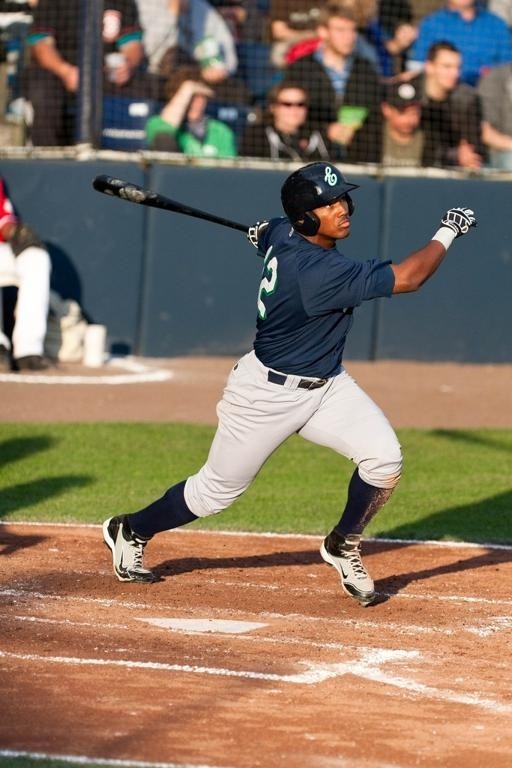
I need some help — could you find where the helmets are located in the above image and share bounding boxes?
[281,161,360,237]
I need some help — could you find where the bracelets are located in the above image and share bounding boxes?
[430,228,457,252]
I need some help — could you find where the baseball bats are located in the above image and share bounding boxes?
[92,174,251,232]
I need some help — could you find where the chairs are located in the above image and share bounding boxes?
[6,38,276,152]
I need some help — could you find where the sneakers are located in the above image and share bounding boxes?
[102,513,155,583]
[320,528,375,597]
[1,344,53,372]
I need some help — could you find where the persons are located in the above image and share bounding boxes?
[0,0,80,147]
[0,177,54,369]
[100,161,478,607]
[101,0,512,171]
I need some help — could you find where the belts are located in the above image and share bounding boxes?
[267,369,328,390]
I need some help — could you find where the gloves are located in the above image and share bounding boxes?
[247,219,269,248]
[441,206,477,239]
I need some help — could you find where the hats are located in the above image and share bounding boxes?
[386,83,429,111]
